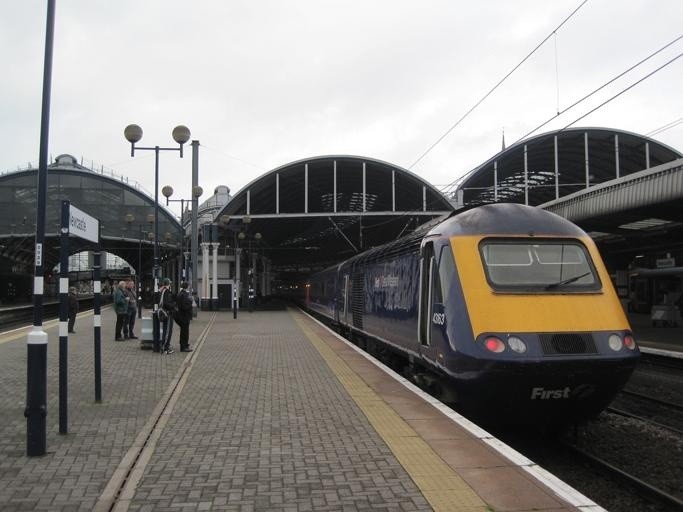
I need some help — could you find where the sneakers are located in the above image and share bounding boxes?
[115,333,138,341]
[162,345,195,354]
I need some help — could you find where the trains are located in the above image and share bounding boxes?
[269,203,640,430]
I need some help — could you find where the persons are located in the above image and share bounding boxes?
[629,287,636,314]
[66,286,78,334]
[103,289,106,295]
[153,277,179,355]
[110,286,113,294]
[173,282,195,352]
[125,279,138,340]
[113,280,131,341]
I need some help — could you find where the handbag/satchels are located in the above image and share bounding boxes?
[156,308,169,323]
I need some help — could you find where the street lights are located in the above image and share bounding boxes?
[126,214,155,318]
[123,124,191,355]
[238,232,263,311]
[221,214,251,319]
[161,186,203,289]
[148,232,172,283]
